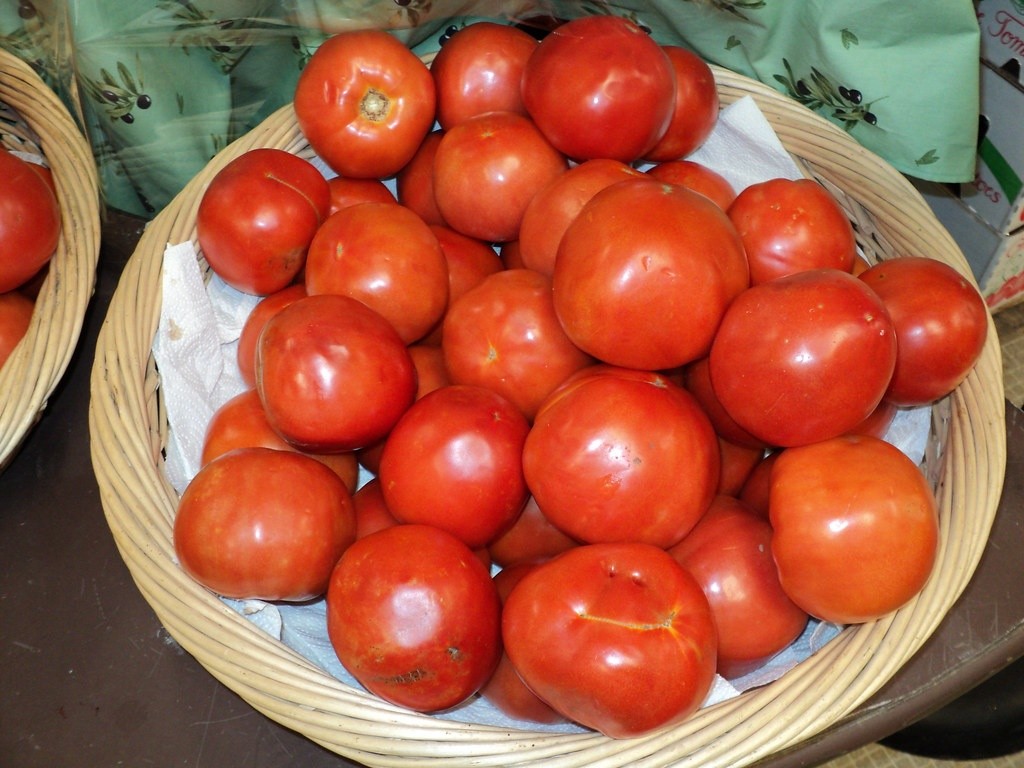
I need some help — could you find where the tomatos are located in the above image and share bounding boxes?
[0,149,62,366]
[175,17,989,732]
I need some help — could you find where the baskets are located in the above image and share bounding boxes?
[1,51,101,465]
[88,49,1006,764]
